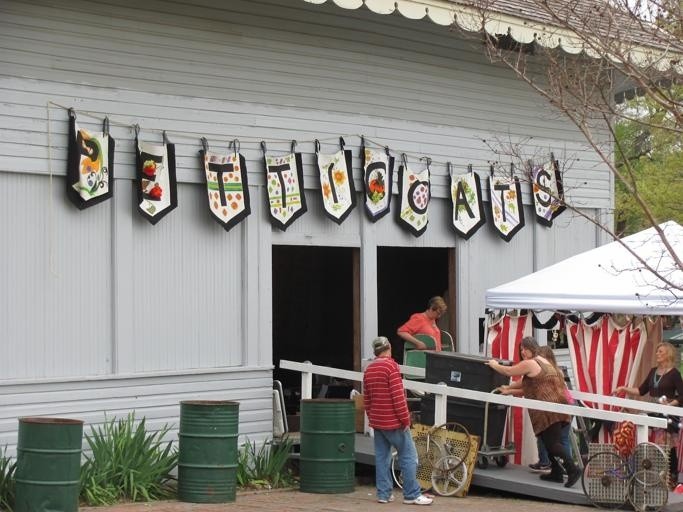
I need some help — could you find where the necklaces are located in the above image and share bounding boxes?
[654,366,666,388]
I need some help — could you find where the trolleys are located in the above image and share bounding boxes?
[471,387,515,469]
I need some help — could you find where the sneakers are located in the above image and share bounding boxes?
[565,465,582,488]
[403,495,433,505]
[540,474,564,483]
[528,462,551,472]
[378,494,395,503]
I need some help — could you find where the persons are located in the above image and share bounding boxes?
[397,296,448,411]
[364,336,433,504]
[609,342,683,491]
[484,336,582,488]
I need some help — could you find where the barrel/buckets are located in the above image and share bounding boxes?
[178,400,239,502]
[14,416,84,512]
[300,398,357,494]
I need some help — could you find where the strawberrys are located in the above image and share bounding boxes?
[143,160,156,177]
[370,172,384,193]
[150,186,162,200]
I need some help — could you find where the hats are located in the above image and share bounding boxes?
[372,336,388,349]
